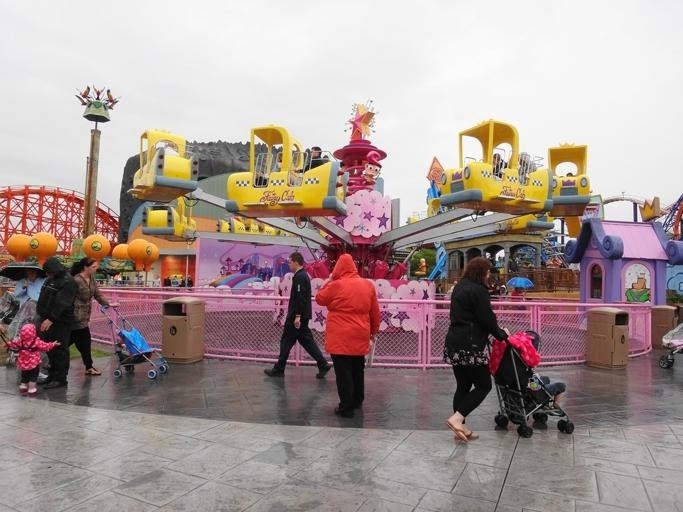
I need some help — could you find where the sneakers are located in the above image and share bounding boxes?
[334,405,354,418]
[264,368,285,377]
[20,388,27,395]
[316,364,332,377]
[29,388,40,397]
[85,368,101,375]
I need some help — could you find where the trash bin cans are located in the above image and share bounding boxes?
[161,296,205,365]
[586,307,630,370]
[651,304,678,349]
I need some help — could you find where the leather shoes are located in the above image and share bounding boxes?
[44,379,67,389]
[38,376,52,383]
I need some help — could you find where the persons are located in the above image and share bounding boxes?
[95,274,194,292]
[68,257,121,376]
[316,254,381,417]
[567,173,573,177]
[492,153,508,179]
[435,255,580,310]
[523,330,567,410]
[0,323,5,336]
[36,257,78,389]
[263,251,331,378]
[13,269,48,308]
[443,257,510,444]
[1,317,61,397]
[293,147,324,173]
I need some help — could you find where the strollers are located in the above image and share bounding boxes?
[487,334,574,438]
[100,304,169,380]
[658,324,683,369]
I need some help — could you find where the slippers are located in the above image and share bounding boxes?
[455,432,478,440]
[446,421,468,442]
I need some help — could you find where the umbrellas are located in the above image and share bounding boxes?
[507,276,534,288]
[0,262,46,280]
